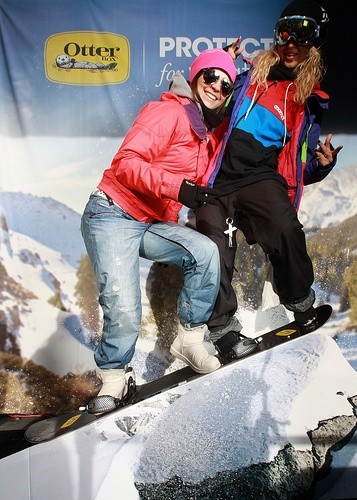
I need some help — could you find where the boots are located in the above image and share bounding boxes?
[170,321,221,374]
[86,364,136,416]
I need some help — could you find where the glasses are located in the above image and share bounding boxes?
[202,68,235,98]
[276,26,315,46]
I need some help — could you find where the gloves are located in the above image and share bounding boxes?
[179,179,222,209]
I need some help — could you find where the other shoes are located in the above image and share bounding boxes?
[293,304,320,334]
[213,331,240,354]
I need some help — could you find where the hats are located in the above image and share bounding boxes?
[274,0,330,42]
[190,47,236,91]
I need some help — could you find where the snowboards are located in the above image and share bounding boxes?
[25,304,333,444]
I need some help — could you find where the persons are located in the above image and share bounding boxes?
[195,0,342,357]
[79,48,237,414]
